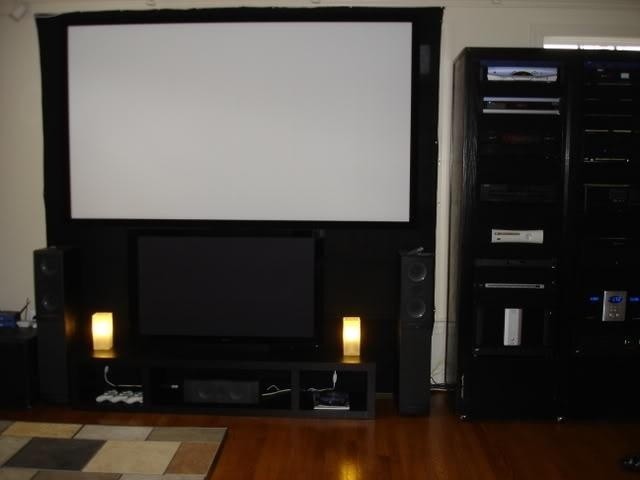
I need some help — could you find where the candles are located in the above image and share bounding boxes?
[342,316,362,357]
[91,312,114,352]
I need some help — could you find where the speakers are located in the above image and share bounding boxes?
[398,249,435,416]
[33,248,70,405]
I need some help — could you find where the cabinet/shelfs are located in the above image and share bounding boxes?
[449,48,640,422]
[97,359,377,420]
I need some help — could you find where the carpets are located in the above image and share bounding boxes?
[1,420,229,479]
